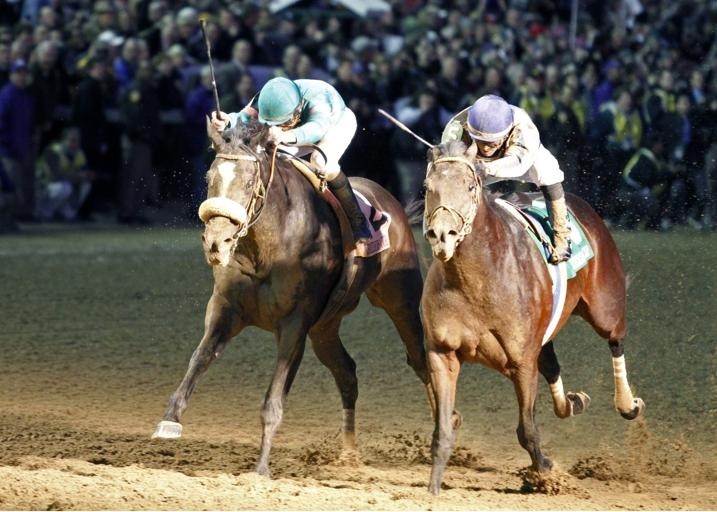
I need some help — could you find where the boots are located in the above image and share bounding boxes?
[545,194,572,264]
[327,169,373,242]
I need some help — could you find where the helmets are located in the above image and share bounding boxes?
[257,76,301,126]
[466,94,514,142]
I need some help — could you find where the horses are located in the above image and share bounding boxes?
[150,112,461,481]
[417,140,646,497]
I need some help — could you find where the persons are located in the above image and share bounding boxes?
[1,1,716,233]
[423,92,580,263]
[209,75,374,246]
